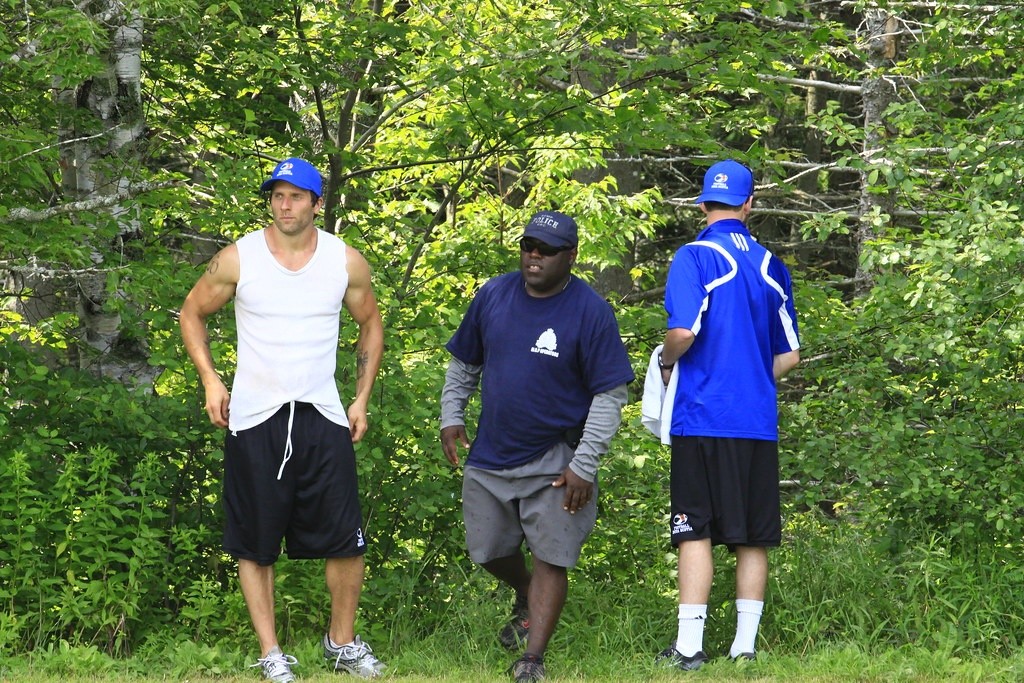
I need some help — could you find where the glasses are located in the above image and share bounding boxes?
[520,238,574,256]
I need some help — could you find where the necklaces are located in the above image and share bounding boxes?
[521,274,571,290]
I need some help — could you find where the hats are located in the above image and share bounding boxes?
[515,211,578,248]
[695,159,753,207]
[260,158,321,197]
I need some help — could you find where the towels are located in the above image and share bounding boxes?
[639,344,680,447]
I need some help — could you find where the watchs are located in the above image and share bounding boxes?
[657,351,676,372]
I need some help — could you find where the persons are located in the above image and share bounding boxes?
[177,158,391,683]
[654,160,802,675]
[438,209,638,683]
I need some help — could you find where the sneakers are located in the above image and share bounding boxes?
[654,647,709,672]
[506,654,545,682]
[728,653,757,668]
[500,596,530,650]
[324,631,386,678]
[260,653,295,682]
